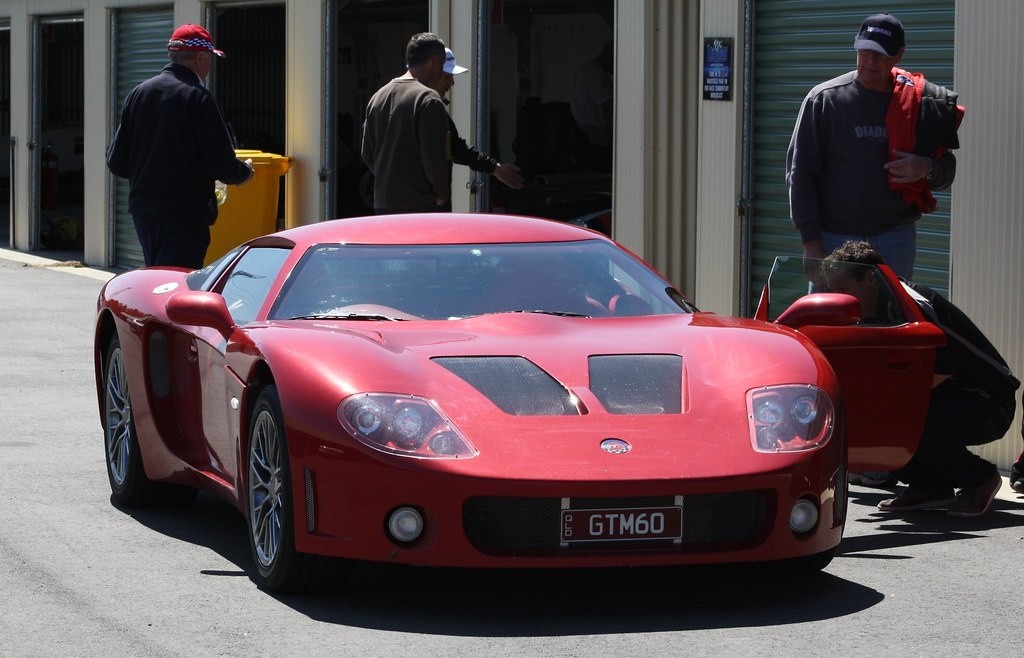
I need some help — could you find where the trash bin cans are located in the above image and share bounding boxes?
[202,150,298,268]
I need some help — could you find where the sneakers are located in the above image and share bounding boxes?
[878,484,954,511]
[947,459,1003,518]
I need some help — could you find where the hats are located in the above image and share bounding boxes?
[443,47,469,76]
[853,12,906,59]
[167,23,227,61]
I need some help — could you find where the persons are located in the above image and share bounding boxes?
[436,45,522,213]
[784,10,956,295]
[361,31,455,217]
[104,23,256,272]
[814,245,1022,520]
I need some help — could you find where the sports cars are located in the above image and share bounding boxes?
[93,212,949,598]
